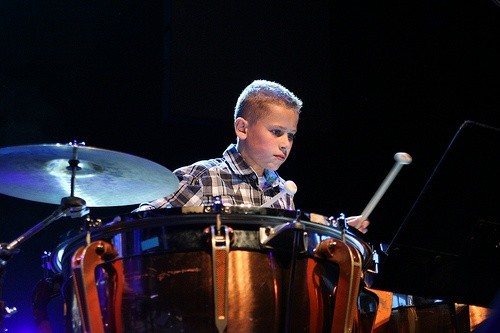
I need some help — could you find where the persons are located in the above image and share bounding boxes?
[129,79,370,232]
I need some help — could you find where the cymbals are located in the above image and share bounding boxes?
[0,145,179,207]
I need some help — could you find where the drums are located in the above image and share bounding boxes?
[50,214,371,333]
